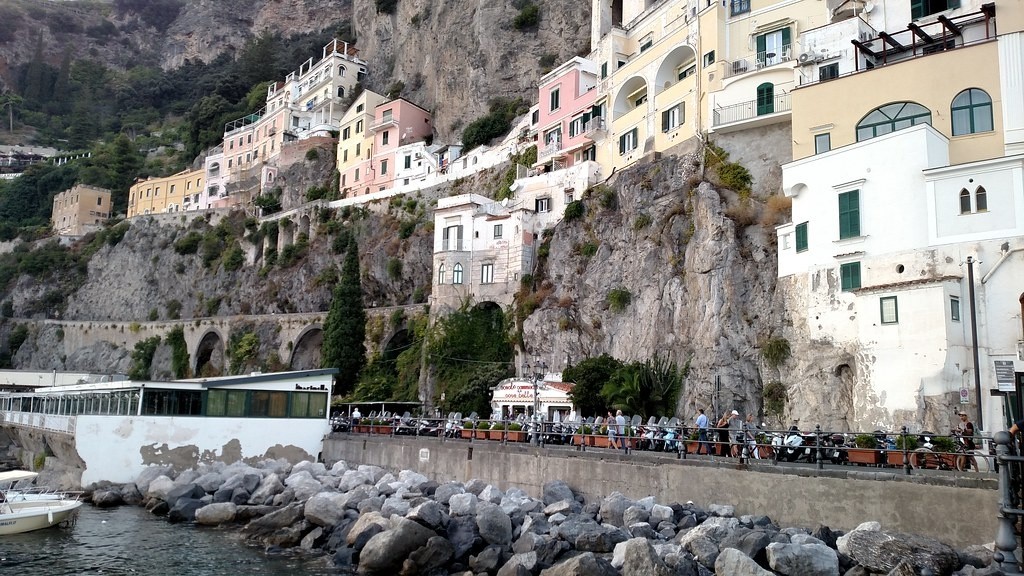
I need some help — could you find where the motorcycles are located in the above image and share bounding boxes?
[329,411,901,465]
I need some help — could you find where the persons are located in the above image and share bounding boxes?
[352,408,361,432]
[954,411,980,472]
[691,408,761,460]
[1010,419,1024,436]
[602,410,625,450]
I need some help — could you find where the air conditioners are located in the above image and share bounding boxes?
[800,51,816,62]
[733,59,748,70]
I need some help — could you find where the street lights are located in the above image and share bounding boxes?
[960,255,984,431]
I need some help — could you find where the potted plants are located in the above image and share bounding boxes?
[616,427,635,447]
[352,419,359,432]
[886,435,923,466]
[474,422,490,439]
[573,426,595,446]
[507,423,528,440]
[751,434,772,457]
[360,418,372,432]
[490,422,505,439]
[686,432,707,453]
[372,418,380,433]
[461,421,472,438]
[595,426,609,446]
[380,420,391,434]
[924,436,958,468]
[847,434,883,463]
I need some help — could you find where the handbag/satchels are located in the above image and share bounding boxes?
[699,429,707,435]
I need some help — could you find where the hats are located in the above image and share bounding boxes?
[732,410,739,416]
[958,411,967,416]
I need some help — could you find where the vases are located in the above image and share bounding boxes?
[716,442,736,455]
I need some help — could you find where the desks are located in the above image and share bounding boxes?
[766,53,776,66]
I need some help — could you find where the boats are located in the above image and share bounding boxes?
[0,486,84,535]
[0,470,38,488]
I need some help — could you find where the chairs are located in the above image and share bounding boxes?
[780,48,792,62]
[754,59,766,70]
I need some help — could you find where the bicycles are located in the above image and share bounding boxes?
[909,430,990,474]
